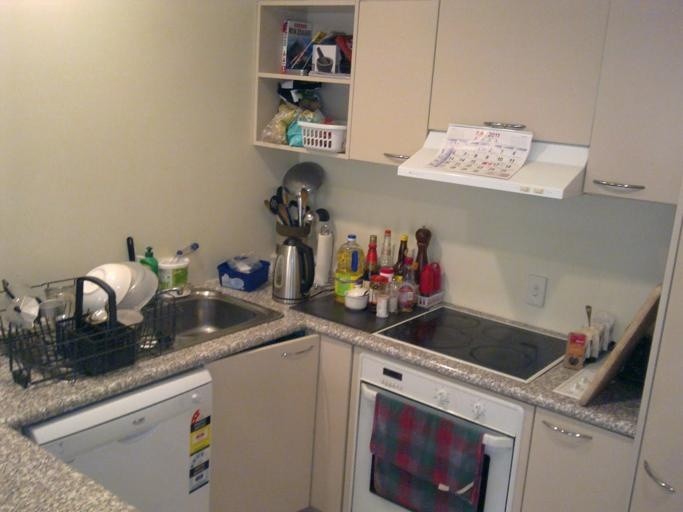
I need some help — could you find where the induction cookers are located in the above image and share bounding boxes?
[372,301,573,384]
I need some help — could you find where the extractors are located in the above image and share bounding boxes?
[397,124,590,202]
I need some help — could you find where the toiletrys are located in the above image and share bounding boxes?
[139,246,159,276]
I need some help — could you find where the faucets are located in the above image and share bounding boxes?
[154,288,184,308]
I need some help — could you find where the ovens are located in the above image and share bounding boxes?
[347,378,522,512]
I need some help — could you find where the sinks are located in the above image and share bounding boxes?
[142,287,284,349]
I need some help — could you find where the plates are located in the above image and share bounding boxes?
[117,310,143,325]
[116,261,160,311]
[82,263,132,324]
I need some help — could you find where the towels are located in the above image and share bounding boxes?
[368,388,482,512]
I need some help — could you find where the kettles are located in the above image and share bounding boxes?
[272,238,314,305]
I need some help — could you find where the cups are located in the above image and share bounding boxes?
[159,257,190,297]
[40,300,65,321]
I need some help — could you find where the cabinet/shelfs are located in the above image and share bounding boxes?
[210,331,321,511]
[512,404,635,511]
[253,0,359,160]
[348,0,441,166]
[427,1,611,147]
[627,210,683,512]
[582,0,683,206]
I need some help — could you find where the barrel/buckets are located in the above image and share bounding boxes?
[334,235,367,302]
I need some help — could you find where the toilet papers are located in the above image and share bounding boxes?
[314,233,333,287]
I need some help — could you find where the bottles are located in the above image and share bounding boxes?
[344,226,442,317]
[179,244,198,257]
[170,251,182,265]
[566,305,614,370]
[314,225,335,288]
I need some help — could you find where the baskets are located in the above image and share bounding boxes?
[297,120,347,153]
[0,279,175,387]
